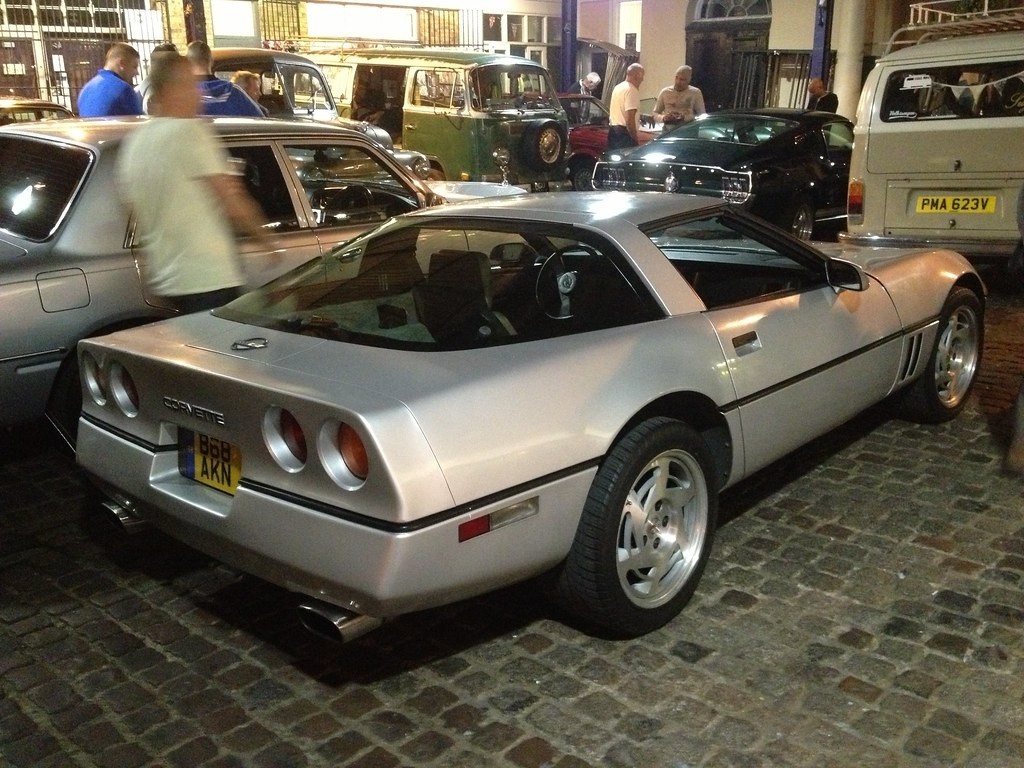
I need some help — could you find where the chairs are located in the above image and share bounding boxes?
[425,247,522,342]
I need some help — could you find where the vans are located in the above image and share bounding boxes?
[294,49,575,192]
[839,30,1024,259]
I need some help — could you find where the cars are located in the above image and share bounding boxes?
[1,101,74,127]
[75,192,987,645]
[1,116,451,429]
[591,107,854,241]
[555,94,660,193]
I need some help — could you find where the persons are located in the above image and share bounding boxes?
[77,43,143,118]
[141,43,179,114]
[184,40,266,118]
[111,51,286,320]
[230,70,270,117]
[806,78,839,145]
[563,73,601,125]
[607,63,645,150]
[354,72,402,144]
[653,65,706,134]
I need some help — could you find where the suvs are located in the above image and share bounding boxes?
[197,48,430,181]
